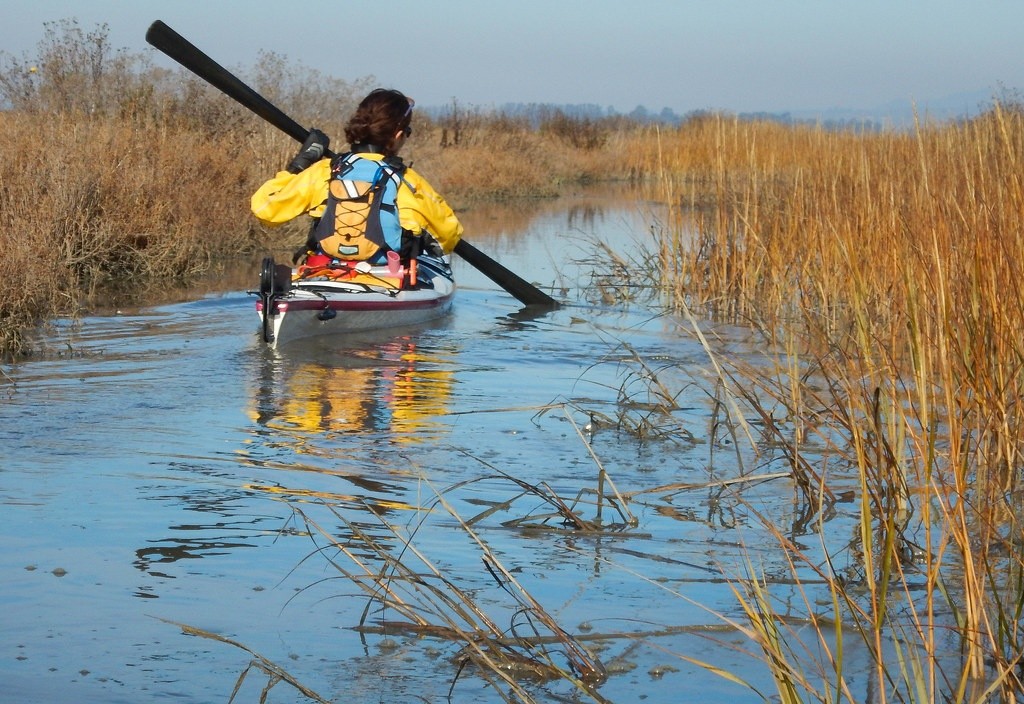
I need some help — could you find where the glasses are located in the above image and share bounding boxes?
[404,96,416,117]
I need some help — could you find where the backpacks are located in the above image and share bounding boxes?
[314,152,406,265]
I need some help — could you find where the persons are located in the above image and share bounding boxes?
[250,88,464,288]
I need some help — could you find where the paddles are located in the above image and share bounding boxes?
[141,16,564,310]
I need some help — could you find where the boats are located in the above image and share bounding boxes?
[245,232,455,350]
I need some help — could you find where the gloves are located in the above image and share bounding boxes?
[287,130,331,174]
